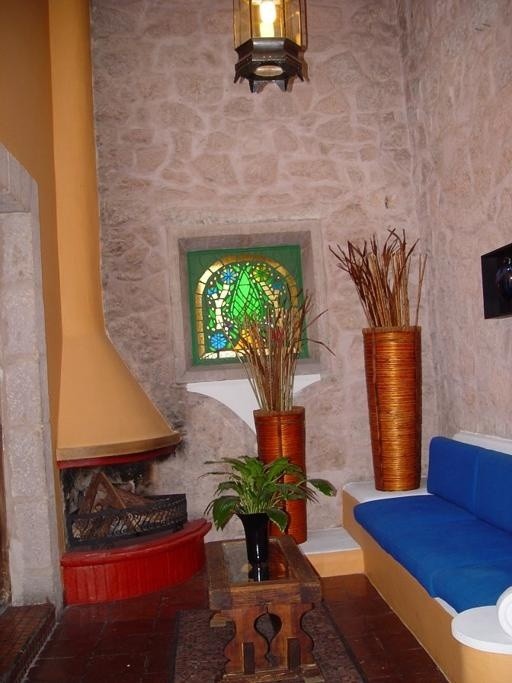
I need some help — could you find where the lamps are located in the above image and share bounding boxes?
[229,0,310,93]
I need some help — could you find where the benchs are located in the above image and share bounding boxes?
[333,430,512,681]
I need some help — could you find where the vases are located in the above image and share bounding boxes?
[362,324,423,490]
[253,407,307,543]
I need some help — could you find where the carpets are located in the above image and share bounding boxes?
[173,596,365,681]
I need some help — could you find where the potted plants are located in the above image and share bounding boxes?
[201,451,338,565]
